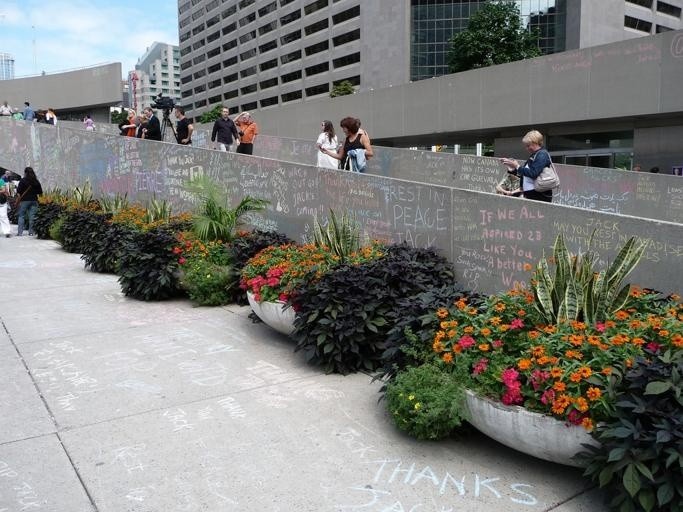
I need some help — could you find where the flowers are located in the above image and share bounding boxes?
[434,283,682,434]
[240,239,394,314]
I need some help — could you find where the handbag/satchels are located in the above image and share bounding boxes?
[534,150,561,192]
[15,197,20,208]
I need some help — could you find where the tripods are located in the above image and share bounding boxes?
[160,109,178,143]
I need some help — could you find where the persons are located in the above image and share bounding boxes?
[15,167,42,236]
[174,105,194,147]
[0,170,12,237]
[499,129,552,203]
[495,160,523,198]
[83,114,93,131]
[211,106,241,152]
[316,117,374,173]
[0,101,57,125]
[121,107,161,141]
[232,112,258,155]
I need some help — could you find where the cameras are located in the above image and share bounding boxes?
[239,131,244,136]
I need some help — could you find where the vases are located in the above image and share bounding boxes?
[447,381,603,472]
[246,291,295,336]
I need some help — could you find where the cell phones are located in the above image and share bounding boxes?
[319,145,324,153]
